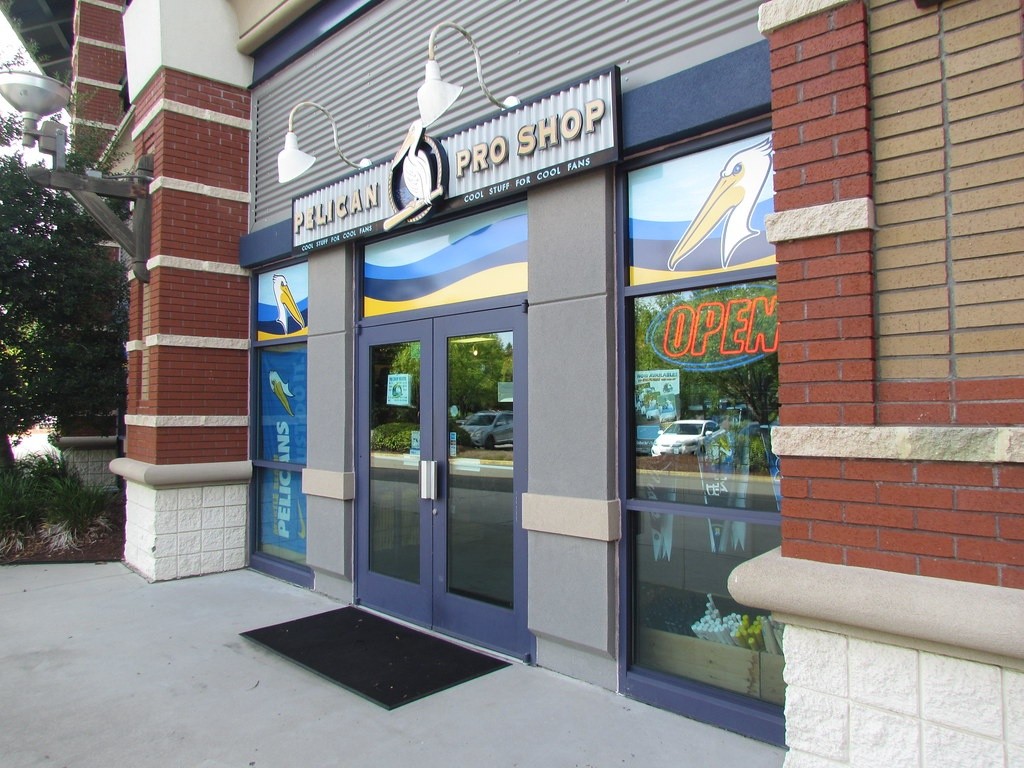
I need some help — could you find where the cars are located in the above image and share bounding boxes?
[456,409,513,450]
[636,424,661,456]
[651,420,725,457]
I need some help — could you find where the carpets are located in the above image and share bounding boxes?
[238,606,513,711]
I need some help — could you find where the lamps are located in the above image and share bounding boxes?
[416,22,521,128]
[277,102,371,184]
